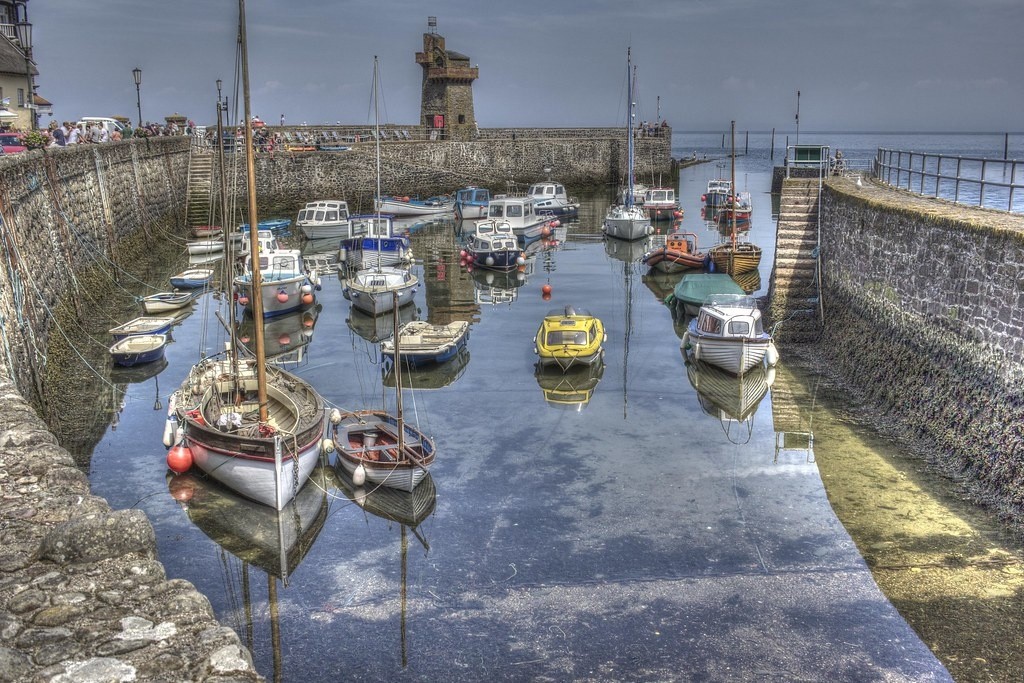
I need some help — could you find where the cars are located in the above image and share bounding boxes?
[0,132,29,154]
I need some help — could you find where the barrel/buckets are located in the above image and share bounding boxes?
[363,432,378,447]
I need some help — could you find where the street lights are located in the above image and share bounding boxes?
[133,67,141,127]
[152,377,162,411]
[216,78,222,102]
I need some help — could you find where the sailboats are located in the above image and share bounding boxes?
[707,119,763,276]
[715,268,762,296]
[339,57,419,314]
[604,233,654,416]
[172,463,328,683]
[604,45,652,242]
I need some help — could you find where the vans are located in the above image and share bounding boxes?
[78,116,124,137]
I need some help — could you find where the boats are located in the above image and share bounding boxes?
[339,212,409,269]
[533,304,605,368]
[347,301,420,343]
[331,285,437,494]
[240,218,290,231]
[110,335,167,368]
[467,218,523,272]
[288,145,316,151]
[108,317,172,341]
[640,187,681,219]
[320,146,347,151]
[702,179,733,207]
[524,182,579,216]
[296,198,369,239]
[380,346,469,390]
[684,357,767,446]
[468,265,525,311]
[110,355,167,382]
[190,225,223,238]
[237,231,279,261]
[641,231,707,274]
[170,0,325,516]
[485,196,558,244]
[374,192,453,218]
[169,267,212,291]
[186,240,222,256]
[716,189,752,220]
[683,301,776,379]
[457,187,495,218]
[617,184,649,207]
[674,273,747,320]
[141,291,190,315]
[231,248,313,317]
[331,455,437,671]
[534,365,603,412]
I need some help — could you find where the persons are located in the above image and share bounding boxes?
[146,120,195,136]
[280,114,285,125]
[238,116,268,144]
[46,118,133,146]
